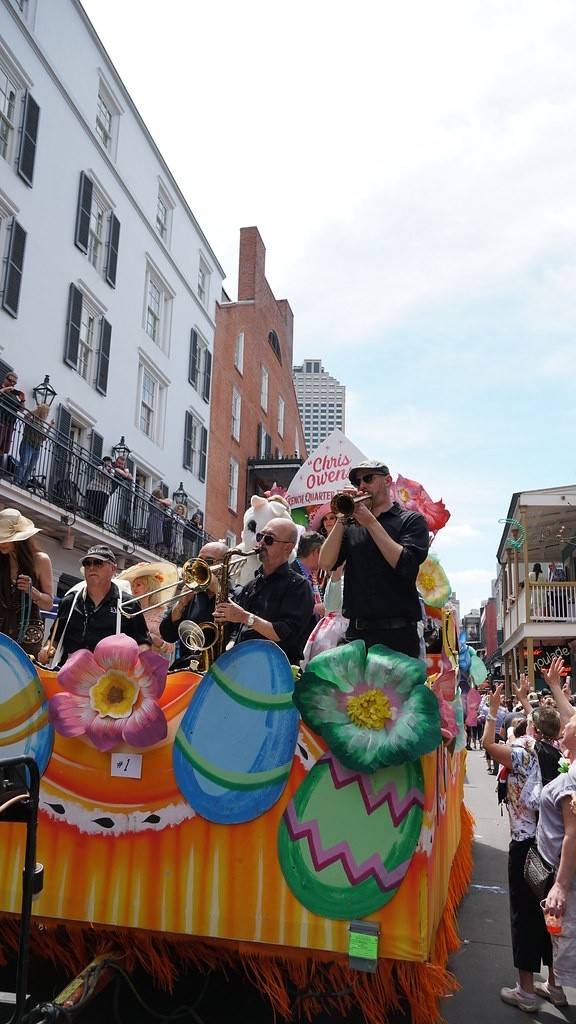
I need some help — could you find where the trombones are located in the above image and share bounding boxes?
[118,557,248,619]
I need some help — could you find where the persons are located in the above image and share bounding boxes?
[0,372,26,476]
[38,508,325,680]
[309,460,429,663]
[12,404,55,489]
[87,456,133,527]
[465,657,576,1013]
[509,561,567,622]
[147,489,202,567]
[0,507,55,663]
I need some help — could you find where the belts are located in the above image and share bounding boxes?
[350,616,413,630]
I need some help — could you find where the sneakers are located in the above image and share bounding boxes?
[533,980,568,1006]
[499,982,537,1013]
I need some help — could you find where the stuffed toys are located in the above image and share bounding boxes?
[230,495,307,595]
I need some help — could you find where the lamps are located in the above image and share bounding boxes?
[32,375,57,407]
[116,558,125,570]
[173,482,188,504]
[112,435,131,460]
[62,534,74,550]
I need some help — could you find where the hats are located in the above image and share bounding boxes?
[79,545,116,566]
[348,459,389,482]
[0,508,43,543]
[309,502,336,531]
[114,561,178,608]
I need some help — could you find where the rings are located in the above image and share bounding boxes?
[222,612,225,617]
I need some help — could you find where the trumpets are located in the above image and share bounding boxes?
[330,491,373,527]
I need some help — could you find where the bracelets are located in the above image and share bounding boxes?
[177,600,185,613]
[158,642,165,648]
[21,399,25,402]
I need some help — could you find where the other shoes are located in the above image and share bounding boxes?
[465,742,499,775]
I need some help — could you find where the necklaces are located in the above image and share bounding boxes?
[295,558,324,604]
[17,576,33,643]
[498,519,525,550]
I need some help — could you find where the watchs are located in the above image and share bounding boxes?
[245,613,255,628]
[486,714,497,722]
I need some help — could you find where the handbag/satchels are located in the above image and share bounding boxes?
[523,848,554,895]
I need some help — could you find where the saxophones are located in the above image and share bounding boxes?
[190,547,260,676]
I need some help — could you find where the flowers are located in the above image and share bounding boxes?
[415,554,452,608]
[390,473,451,536]
[426,654,461,746]
[49,632,170,752]
[292,640,444,770]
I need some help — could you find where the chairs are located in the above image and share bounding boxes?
[53,478,90,518]
[8,455,47,498]
[121,513,151,549]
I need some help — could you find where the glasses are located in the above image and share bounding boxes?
[6,377,19,386]
[352,472,383,487]
[81,559,106,568]
[256,532,296,547]
[204,559,223,565]
